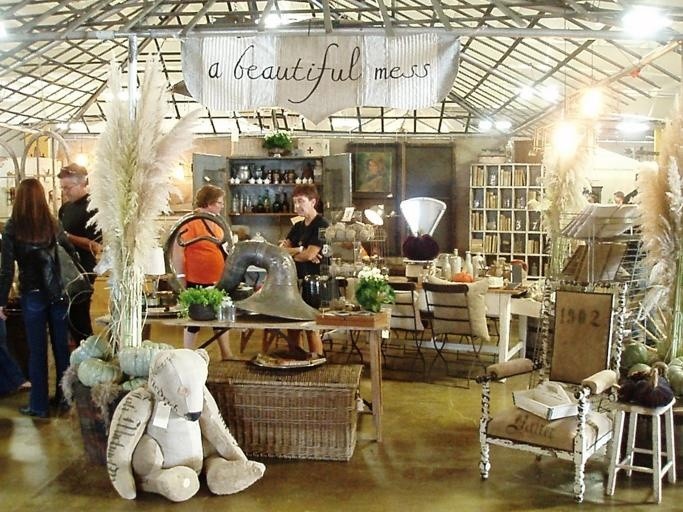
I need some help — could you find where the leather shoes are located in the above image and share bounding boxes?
[19,405,50,418]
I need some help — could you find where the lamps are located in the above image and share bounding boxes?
[363,204,400,226]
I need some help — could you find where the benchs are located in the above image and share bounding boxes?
[71,360,364,464]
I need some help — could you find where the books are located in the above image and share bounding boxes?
[469,166,548,277]
[560,205,641,282]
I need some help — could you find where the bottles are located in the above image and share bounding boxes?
[216,297,236,324]
[330,256,371,279]
[226,160,322,213]
[440,248,485,280]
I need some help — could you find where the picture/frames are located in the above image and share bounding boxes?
[344,139,398,200]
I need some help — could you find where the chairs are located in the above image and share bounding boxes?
[421,280,486,388]
[475,275,630,503]
[387,281,425,372]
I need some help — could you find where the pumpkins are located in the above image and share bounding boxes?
[618,340,682,408]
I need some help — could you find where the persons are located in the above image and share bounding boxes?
[56,165,102,347]
[611,191,627,205]
[280,185,330,361]
[0,180,74,418]
[357,150,390,192]
[0,315,27,398]
[173,186,240,362]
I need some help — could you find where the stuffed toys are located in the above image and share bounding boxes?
[106,349,266,501]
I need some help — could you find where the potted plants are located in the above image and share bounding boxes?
[621,94,681,471]
[61,39,208,461]
[352,277,391,313]
[178,282,227,321]
[261,129,294,155]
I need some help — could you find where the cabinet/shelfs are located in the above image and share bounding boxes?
[528,210,648,386]
[318,223,390,278]
[466,162,551,281]
[221,155,327,220]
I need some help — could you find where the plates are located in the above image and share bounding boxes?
[250,353,326,371]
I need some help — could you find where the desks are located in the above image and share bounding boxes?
[387,272,542,365]
[92,299,391,445]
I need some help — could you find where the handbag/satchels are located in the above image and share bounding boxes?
[30,235,94,306]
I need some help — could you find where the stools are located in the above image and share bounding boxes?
[605,396,677,505]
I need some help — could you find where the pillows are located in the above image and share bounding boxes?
[427,271,491,343]
[389,291,425,332]
